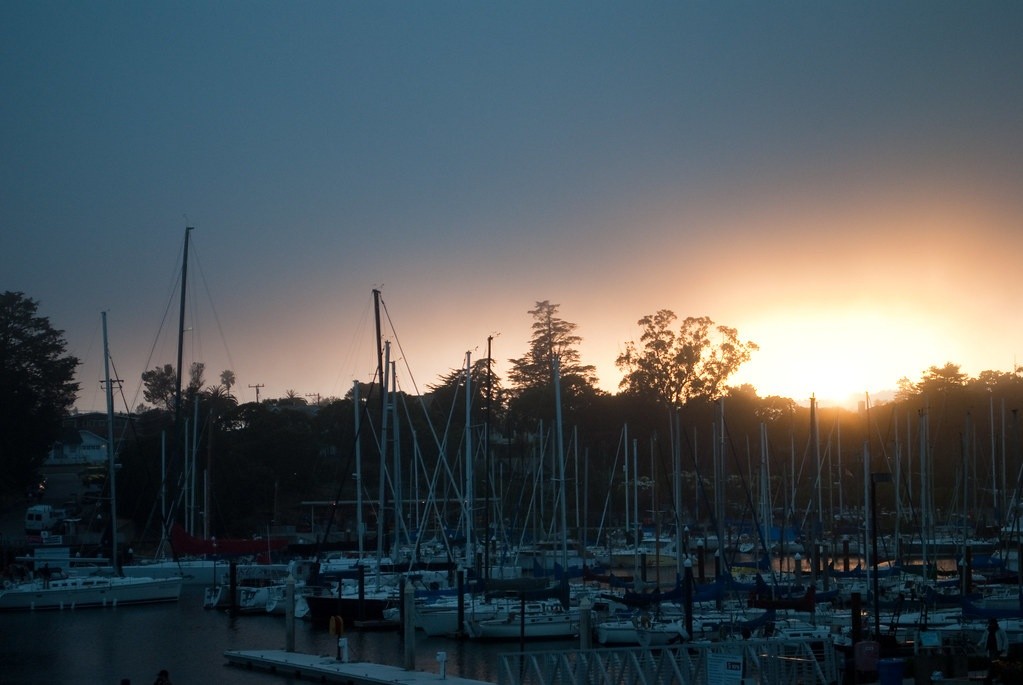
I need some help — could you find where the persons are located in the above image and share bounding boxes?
[121,679,130,685]
[976,618,1008,682]
[116,548,124,576]
[154,670,172,685]
[8,565,26,581]
[43,564,51,589]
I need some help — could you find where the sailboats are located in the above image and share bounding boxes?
[1,224,1023,671]
